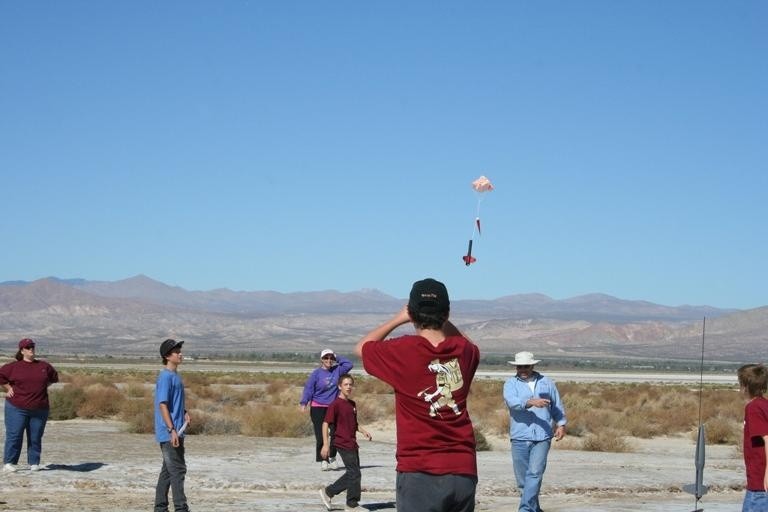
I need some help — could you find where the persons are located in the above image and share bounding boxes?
[503,351,567,512]
[318,373,372,511]
[0,338,59,472]
[738,363,767,512]
[355,278,481,512]
[154,339,190,512]
[299,349,354,471]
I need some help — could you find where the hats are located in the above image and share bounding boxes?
[160,338,184,356]
[320,349,333,357]
[407,276,449,313]
[507,351,543,366]
[18,339,35,348]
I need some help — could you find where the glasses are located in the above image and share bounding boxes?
[169,350,181,354]
[515,365,530,368]
[23,345,34,349]
[324,356,335,361]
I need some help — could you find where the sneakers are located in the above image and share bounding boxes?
[329,457,338,470]
[324,461,329,470]
[319,486,335,509]
[5,464,18,472]
[345,504,370,511]
[29,463,41,471]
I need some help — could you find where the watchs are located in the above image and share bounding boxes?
[167,425,174,433]
